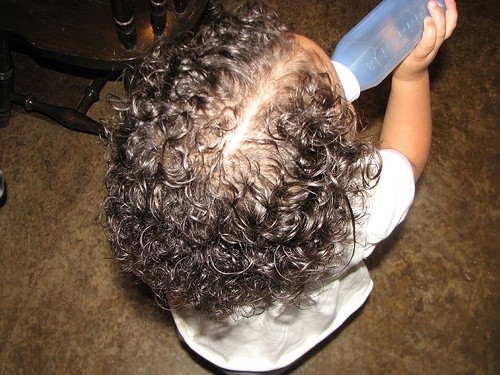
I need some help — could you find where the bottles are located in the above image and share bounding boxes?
[330,0,446,102]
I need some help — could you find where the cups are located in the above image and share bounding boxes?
[111,0,134,26]
[151,0,167,17]
[174,0,187,13]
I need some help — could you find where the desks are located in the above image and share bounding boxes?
[0,0,210,139]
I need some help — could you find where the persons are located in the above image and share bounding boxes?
[94,0,459,375]
